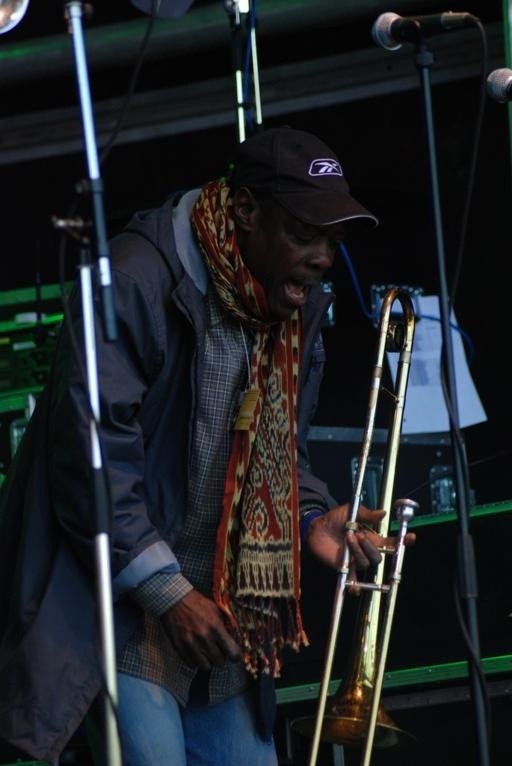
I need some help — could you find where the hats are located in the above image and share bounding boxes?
[231,126,379,229]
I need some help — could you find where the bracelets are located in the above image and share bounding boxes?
[298,510,323,544]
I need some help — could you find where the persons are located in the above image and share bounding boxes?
[3,127,415,766]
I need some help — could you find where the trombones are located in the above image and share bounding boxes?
[292,287,420,764]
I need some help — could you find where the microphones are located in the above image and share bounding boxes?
[486,67,512,103]
[373,11,479,51]
